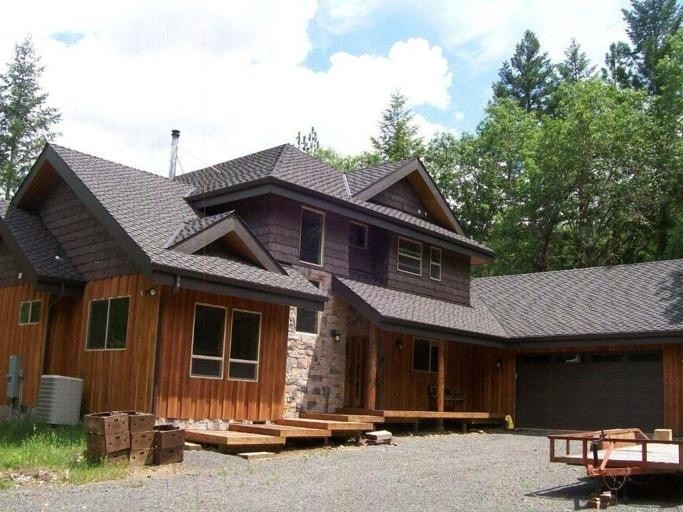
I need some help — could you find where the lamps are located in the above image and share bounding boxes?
[332,328,341,343]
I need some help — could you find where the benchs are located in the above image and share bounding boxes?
[428,384,465,411]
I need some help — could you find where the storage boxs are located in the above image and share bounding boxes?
[83,410,186,467]
